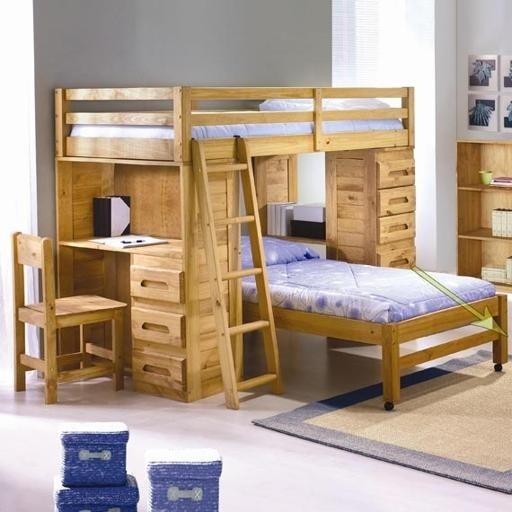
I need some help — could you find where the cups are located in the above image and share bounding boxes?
[479,170,493,185]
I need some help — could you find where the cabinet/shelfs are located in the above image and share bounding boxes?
[456,141,512,296]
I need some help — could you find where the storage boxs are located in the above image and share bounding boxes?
[144,447,223,512]
[59,421,130,486]
[52,473,140,512]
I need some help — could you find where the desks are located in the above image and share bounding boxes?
[59,233,242,404]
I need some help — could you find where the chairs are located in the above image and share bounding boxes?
[11,231,129,404]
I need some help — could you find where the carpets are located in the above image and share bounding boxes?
[253,343,511,496]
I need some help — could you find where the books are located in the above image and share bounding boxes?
[492,208,512,239]
[489,176,512,188]
[91,195,169,249]
[481,256,512,286]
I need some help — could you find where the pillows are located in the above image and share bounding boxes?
[259,98,392,114]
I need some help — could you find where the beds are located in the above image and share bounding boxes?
[52,86,419,405]
[240,234,509,411]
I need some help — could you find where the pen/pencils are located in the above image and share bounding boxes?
[121,240,145,243]
